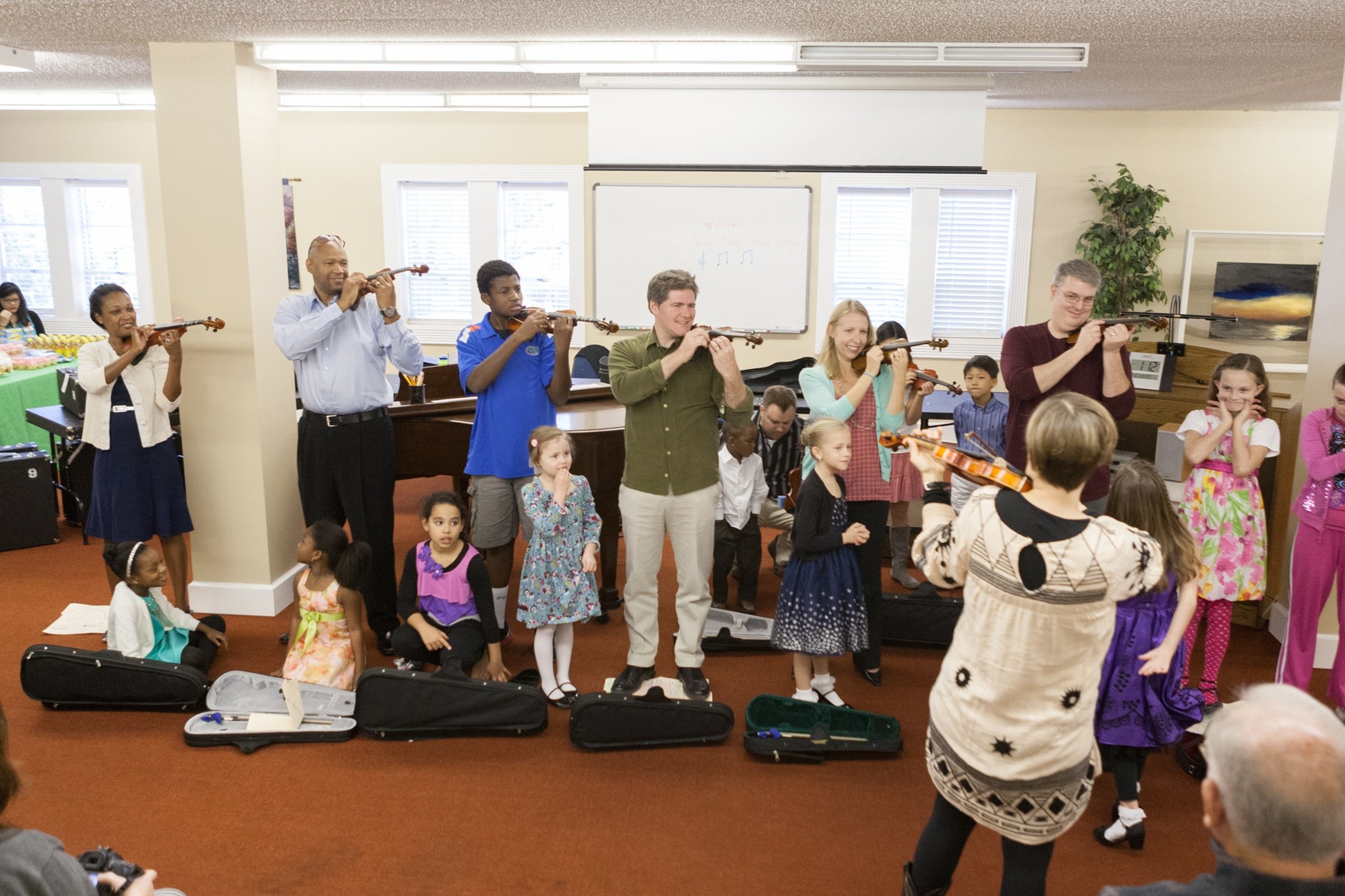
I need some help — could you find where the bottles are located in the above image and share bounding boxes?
[27,333,110,358]
[438,354,448,366]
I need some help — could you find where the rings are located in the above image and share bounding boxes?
[715,348,720,352]
[895,359,898,361]
[165,339,171,344]
[1110,332,1116,335]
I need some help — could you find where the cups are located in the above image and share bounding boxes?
[409,384,426,405]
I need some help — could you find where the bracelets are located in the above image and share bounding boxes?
[863,372,875,379]
[0,324,6,327]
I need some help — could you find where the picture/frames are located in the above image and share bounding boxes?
[1175,229,1326,374]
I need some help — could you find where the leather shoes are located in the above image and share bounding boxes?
[611,665,656,693]
[677,667,710,699]
[278,633,289,645]
[377,618,400,656]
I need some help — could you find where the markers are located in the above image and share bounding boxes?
[755,330,770,332]
[625,327,653,330]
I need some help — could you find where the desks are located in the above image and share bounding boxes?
[0,360,78,456]
[25,404,88,546]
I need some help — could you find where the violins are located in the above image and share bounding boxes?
[783,439,805,512]
[878,430,1033,495]
[1066,317,1170,346]
[679,323,764,349]
[906,360,963,398]
[338,263,429,311]
[506,307,621,336]
[121,316,226,366]
[851,337,949,377]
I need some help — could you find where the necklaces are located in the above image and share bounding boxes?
[835,377,875,431]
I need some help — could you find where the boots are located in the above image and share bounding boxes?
[902,861,954,896]
[889,525,921,589]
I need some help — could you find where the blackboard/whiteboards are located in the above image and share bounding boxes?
[592,182,814,334]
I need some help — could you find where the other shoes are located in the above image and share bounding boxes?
[498,619,511,640]
[1197,677,1223,711]
[731,560,741,579]
[713,602,729,610]
[738,598,757,614]
[767,534,785,577]
[540,686,570,710]
[559,682,580,704]
[863,669,883,686]
[814,688,852,710]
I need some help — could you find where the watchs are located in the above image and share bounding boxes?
[378,306,398,318]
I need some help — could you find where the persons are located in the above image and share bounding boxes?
[270,236,425,656]
[898,392,1165,896]
[0,700,187,896]
[76,282,195,615]
[796,298,934,589]
[711,382,808,613]
[458,259,574,639]
[607,269,755,700]
[0,282,45,344]
[1093,353,1345,896]
[1000,258,1137,518]
[516,424,603,712]
[102,541,229,674]
[390,491,512,683]
[950,353,1009,516]
[270,521,374,691]
[769,417,870,708]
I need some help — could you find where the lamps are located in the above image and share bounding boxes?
[796,42,1090,73]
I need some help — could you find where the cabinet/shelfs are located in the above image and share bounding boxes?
[1124,341,1302,629]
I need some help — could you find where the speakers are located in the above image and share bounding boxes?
[1155,422,1195,482]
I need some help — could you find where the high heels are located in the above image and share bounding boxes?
[1092,803,1145,851]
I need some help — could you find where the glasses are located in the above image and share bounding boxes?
[3,298,20,304]
[1056,286,1097,306]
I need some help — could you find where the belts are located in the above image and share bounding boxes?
[302,407,390,428]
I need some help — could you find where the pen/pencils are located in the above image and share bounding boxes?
[402,372,425,386]
